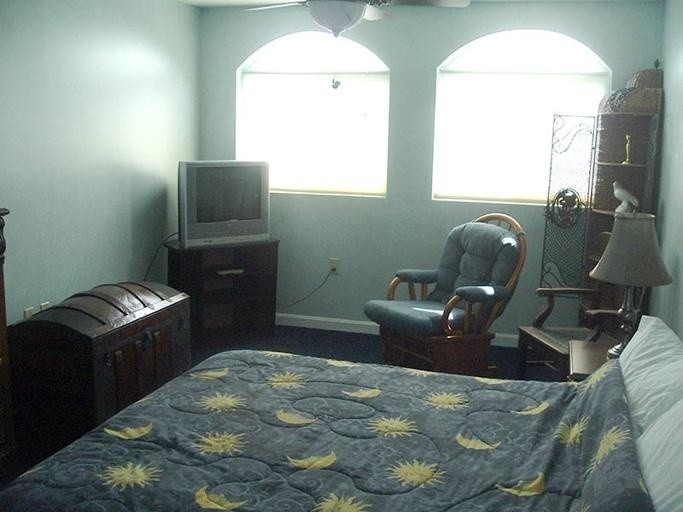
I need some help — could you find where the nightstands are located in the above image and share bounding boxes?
[568,338,622,380]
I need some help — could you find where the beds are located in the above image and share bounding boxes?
[0,348,682,512]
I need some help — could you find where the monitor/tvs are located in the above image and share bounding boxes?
[177,160,270,247]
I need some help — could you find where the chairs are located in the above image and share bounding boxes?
[515,276,649,378]
[363,211,526,374]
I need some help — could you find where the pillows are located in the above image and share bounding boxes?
[615,314,683,511]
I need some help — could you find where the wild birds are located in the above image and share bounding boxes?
[611,180,640,209]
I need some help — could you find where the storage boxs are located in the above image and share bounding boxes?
[6,279,191,449]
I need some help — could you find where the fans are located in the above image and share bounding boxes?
[242,0,473,13]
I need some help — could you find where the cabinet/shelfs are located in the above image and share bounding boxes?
[163,236,281,349]
[579,110,663,290]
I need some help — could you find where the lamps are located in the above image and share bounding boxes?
[588,209,677,359]
[304,0,370,39]
